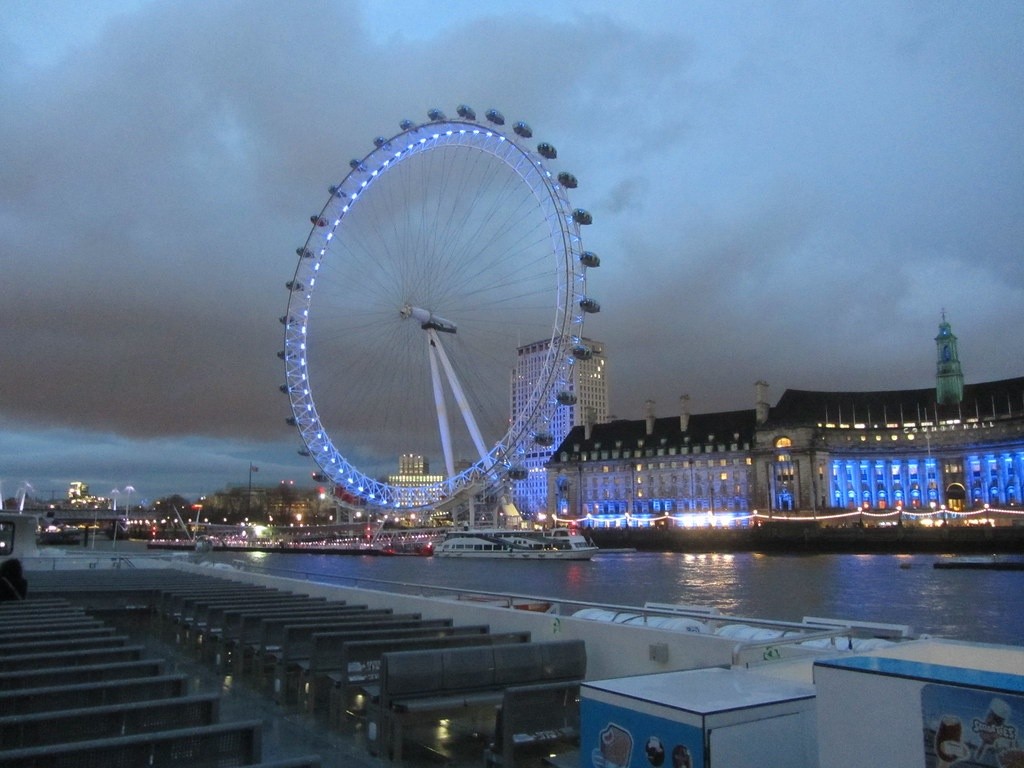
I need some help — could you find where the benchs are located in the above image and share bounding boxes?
[0,569,586,768]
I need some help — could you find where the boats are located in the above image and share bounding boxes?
[433,524,599,560]
